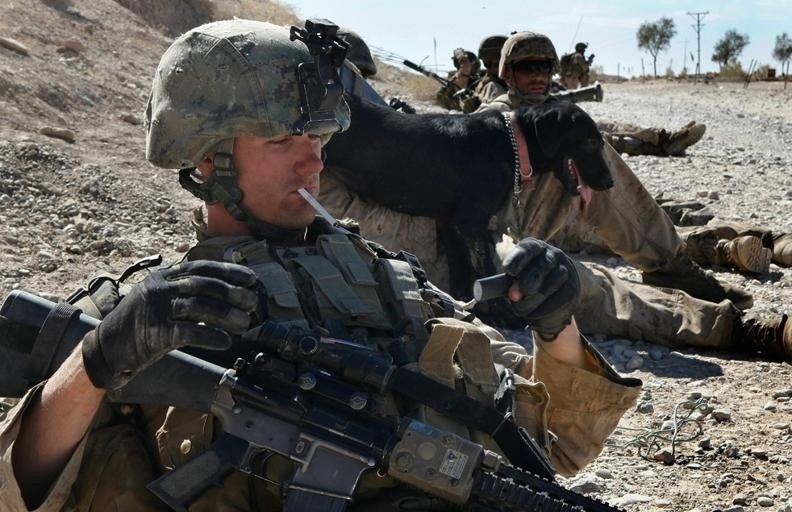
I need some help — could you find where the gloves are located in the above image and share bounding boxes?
[80,259,259,392]
[500,237,582,335]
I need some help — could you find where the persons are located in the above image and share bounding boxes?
[478,29,792,276]
[554,40,595,89]
[436,50,484,111]
[474,34,709,158]
[0,18,645,512]
[317,30,791,366]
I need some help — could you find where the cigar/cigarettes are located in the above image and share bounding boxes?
[296,187,341,230]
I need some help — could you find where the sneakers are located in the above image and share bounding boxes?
[642,253,753,310]
[731,314,792,360]
[715,235,772,274]
[665,119,706,156]
[773,233,792,266]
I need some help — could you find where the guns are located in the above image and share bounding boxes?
[0,289,624,511]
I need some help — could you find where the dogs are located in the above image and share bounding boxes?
[321,87,615,330]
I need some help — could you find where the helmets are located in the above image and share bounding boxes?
[335,31,377,75]
[454,51,477,69]
[144,20,351,170]
[478,36,509,58]
[575,43,587,50]
[497,31,560,79]
